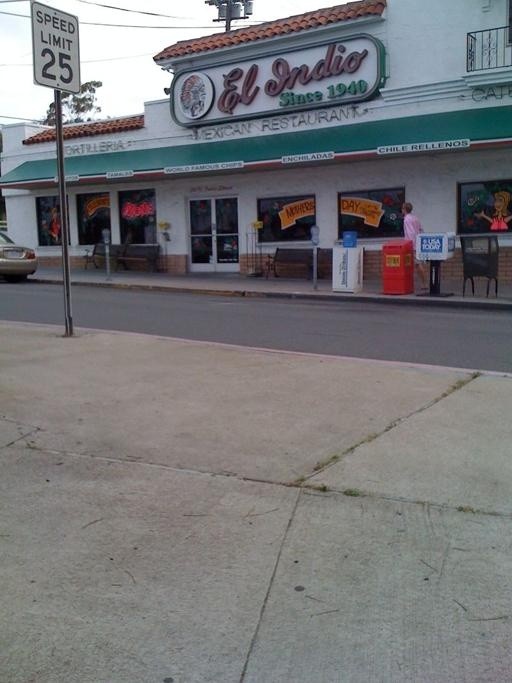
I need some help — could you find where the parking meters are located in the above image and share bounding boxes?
[308,223,320,291]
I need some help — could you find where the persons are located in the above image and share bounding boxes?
[401,203,428,290]
[48,208,62,246]
[474,191,512,230]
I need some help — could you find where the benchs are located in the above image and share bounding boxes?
[264,247,333,281]
[82,242,160,271]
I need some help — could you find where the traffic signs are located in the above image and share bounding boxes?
[29,0,83,97]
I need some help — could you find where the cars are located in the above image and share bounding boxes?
[0,231,38,282]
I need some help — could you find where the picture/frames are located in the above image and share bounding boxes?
[455,176,512,235]
[34,193,72,247]
[117,187,157,246]
[75,191,112,246]
[256,193,317,244]
[336,186,406,241]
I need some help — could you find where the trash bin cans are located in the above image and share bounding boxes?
[382,239,413,295]
